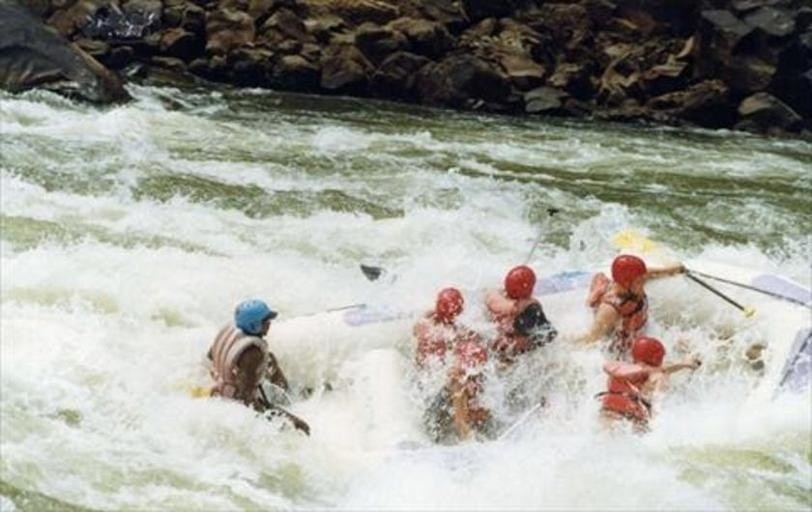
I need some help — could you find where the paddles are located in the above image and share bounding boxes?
[615,232,756,317]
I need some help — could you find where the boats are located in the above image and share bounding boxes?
[141,257,810,471]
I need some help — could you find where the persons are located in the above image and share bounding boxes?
[209,299,278,414]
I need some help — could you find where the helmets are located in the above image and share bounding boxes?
[235,298,277,336]
[505,266,536,299]
[612,255,647,290]
[633,337,665,367]
[455,342,488,367]
[436,288,465,319]
